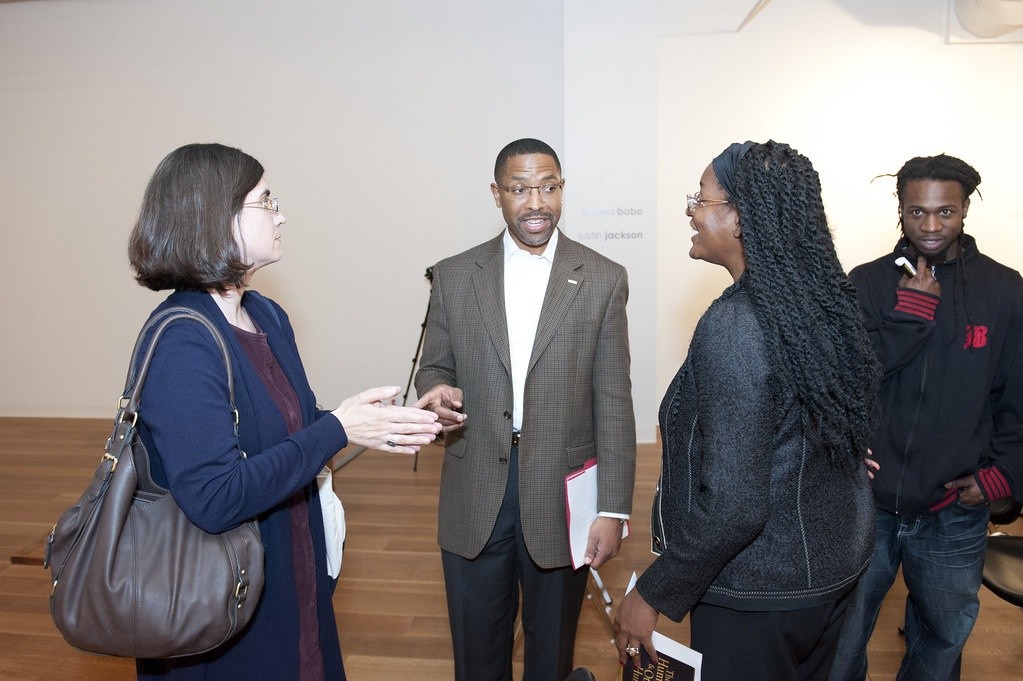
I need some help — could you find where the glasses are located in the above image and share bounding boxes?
[686,192,732,213]
[498,178,565,199]
[240,199,279,213]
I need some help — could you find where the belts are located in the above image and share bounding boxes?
[511,430,521,449]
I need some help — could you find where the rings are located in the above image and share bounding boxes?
[625,647,639,657]
[387,434,396,447]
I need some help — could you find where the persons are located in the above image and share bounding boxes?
[413,139,636,681]
[612,139,885,681]
[123,144,442,681]
[828,154,1023,681]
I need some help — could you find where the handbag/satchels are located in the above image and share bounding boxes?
[44,306,264,659]
